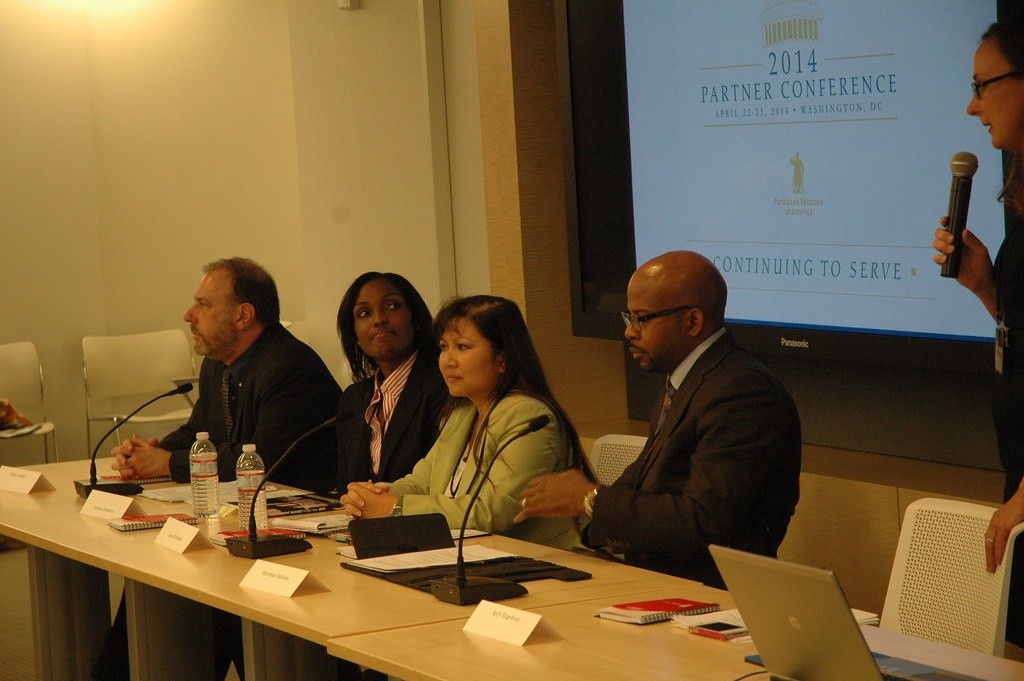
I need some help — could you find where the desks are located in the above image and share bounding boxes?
[327,583,1024,681]
[0,456,704,681]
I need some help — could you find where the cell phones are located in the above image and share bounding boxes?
[690,621,749,640]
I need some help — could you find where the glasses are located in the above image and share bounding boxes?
[620,305,692,333]
[971,68,1023,97]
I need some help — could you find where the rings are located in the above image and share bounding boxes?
[985,537,995,543]
[522,497,527,510]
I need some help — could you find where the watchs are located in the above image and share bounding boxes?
[390,495,404,517]
[583,486,601,518]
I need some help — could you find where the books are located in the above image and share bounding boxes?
[597,599,720,625]
[106,512,198,531]
[100,475,171,485]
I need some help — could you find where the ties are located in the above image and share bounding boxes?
[634,382,675,486]
[221,365,235,444]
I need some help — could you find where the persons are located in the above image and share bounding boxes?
[88,256,344,681]
[513,250,802,592]
[325,272,453,503]
[337,296,595,552]
[934,23,1024,653]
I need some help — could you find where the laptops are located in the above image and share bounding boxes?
[706,544,987,681]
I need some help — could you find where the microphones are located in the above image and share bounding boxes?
[73,383,194,500]
[224,410,354,558]
[431,415,550,607]
[941,151,979,278]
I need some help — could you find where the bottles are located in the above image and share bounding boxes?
[236,444,268,531]
[189,432,219,518]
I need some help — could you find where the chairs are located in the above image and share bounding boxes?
[879,497,1024,657]
[0,341,59,463]
[589,434,649,487]
[82,329,197,459]
